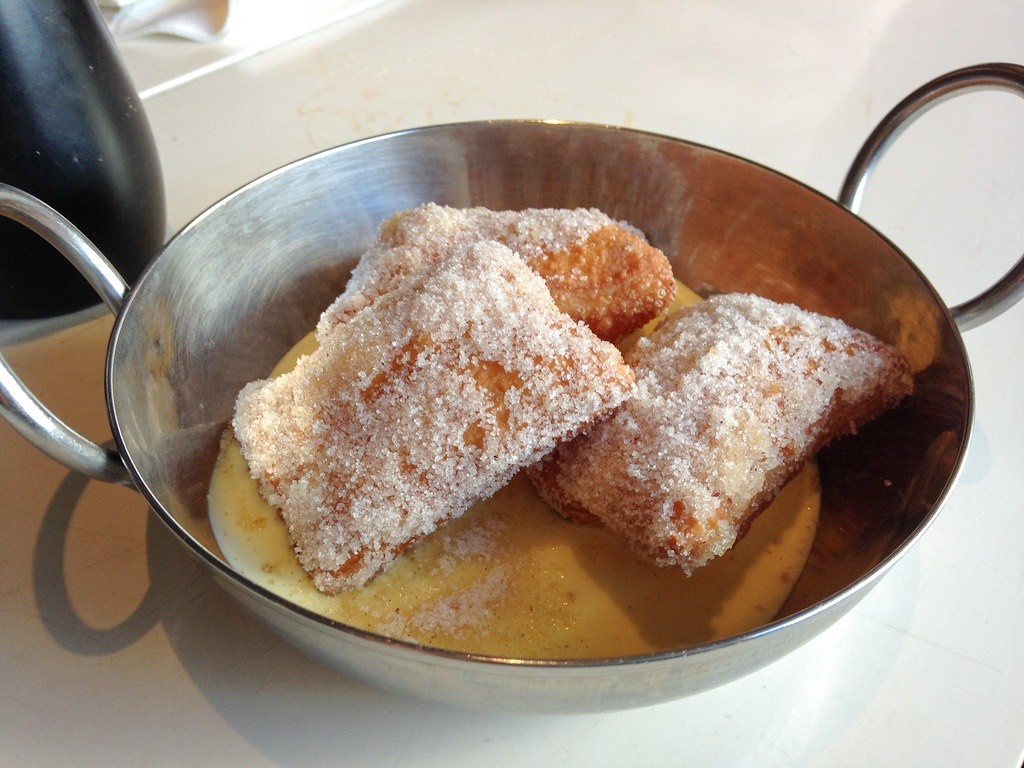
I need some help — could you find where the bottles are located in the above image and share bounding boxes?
[0,0,167,320]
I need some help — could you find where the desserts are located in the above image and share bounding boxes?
[228,197,915,595]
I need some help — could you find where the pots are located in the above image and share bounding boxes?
[0,62,1024,719]
